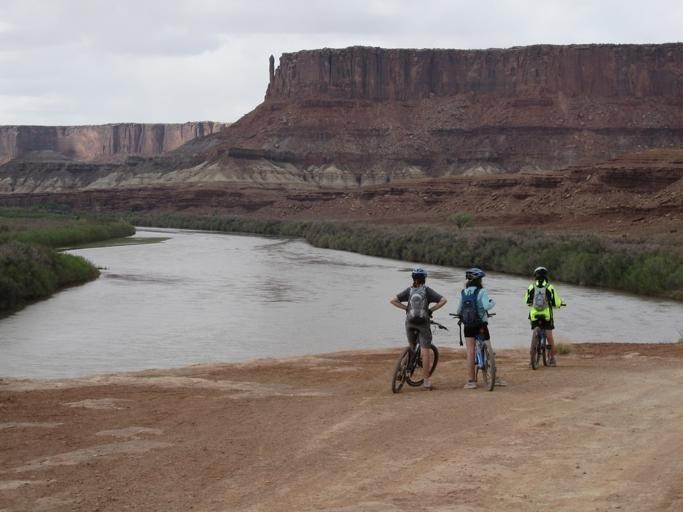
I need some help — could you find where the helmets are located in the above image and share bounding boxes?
[532,266,548,277]
[411,268,427,278]
[464,267,485,281]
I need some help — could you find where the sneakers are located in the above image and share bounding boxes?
[546,358,557,367]
[493,377,508,386]
[463,381,478,389]
[392,366,435,392]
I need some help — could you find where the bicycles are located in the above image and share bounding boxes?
[391,313,496,394]
[528,304,566,370]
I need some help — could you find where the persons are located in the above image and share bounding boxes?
[389,268,448,391]
[457,268,507,390]
[525,265,562,367]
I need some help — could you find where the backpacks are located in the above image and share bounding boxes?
[406,284,429,324]
[532,281,550,312]
[460,287,482,322]
